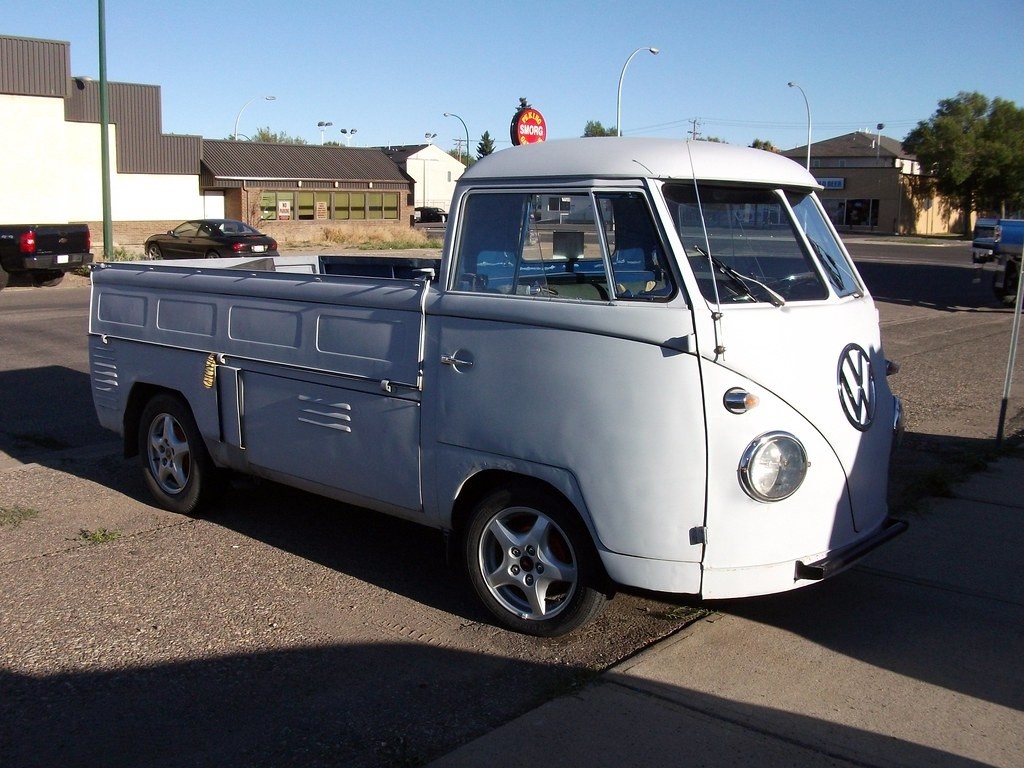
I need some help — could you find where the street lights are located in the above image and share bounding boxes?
[787,81,812,172]
[443,113,469,169]
[618,46,659,136]
[235,95,279,141]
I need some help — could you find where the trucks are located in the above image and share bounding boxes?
[972,218,1024,306]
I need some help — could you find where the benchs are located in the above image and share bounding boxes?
[509,276,666,301]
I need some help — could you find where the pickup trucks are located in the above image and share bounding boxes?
[87,136,916,641]
[0,223,94,290]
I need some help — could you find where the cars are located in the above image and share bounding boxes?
[144,219,279,261]
[415,206,449,223]
